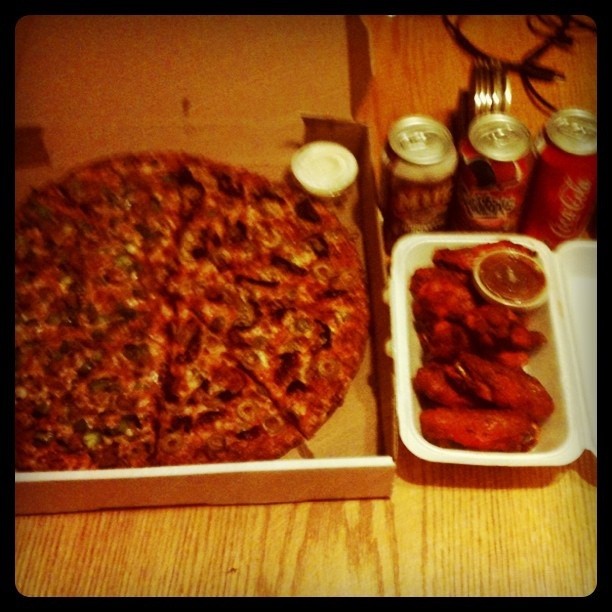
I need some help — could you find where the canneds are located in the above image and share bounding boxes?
[284,141,361,222]
[378,115,458,240]
[526,108,596,247]
[455,112,537,232]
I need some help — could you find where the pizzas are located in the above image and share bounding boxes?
[16,147,371,474]
